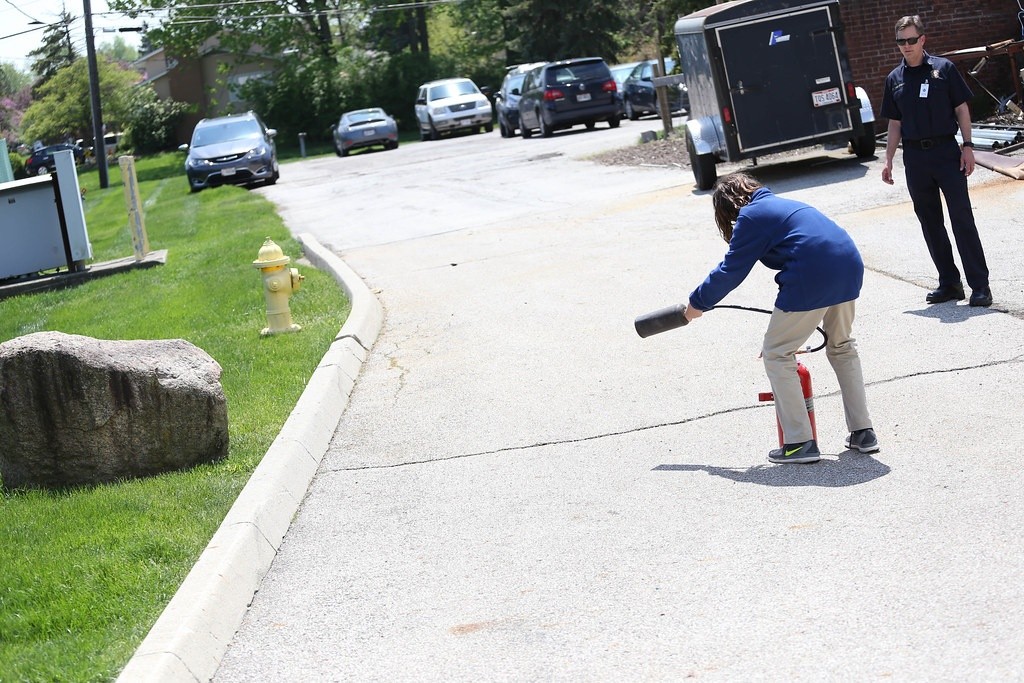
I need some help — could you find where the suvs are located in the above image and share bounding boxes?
[415,78,494,142]
[178,109,280,193]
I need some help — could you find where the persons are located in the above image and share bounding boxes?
[684,173,880,463]
[882,15,993,307]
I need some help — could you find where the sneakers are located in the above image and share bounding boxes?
[845,428,880,453]
[769,439,821,463]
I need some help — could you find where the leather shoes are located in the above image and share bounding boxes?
[926,281,965,303]
[970,286,992,306]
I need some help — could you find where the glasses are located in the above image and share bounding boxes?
[896,34,923,46]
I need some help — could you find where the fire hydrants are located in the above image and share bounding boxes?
[252,236,306,336]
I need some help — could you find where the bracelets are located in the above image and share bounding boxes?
[963,142,974,147]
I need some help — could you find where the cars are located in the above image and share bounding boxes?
[103,132,127,156]
[513,57,627,139]
[620,57,691,121]
[492,62,580,139]
[330,106,399,157]
[606,61,641,94]
[15,142,94,180]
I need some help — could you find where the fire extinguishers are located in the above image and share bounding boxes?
[634,303,828,448]
